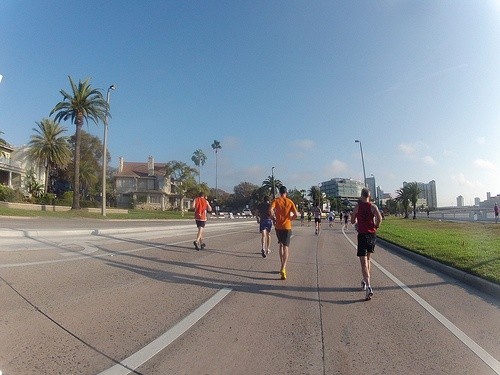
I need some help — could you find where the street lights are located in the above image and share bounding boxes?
[354,139,367,187]
[101,84,117,217]
[271,166,276,201]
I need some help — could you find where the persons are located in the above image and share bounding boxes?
[340,211,350,224]
[351,188,383,300]
[495,203,499,217]
[314,204,321,234]
[328,210,335,227]
[307,209,312,223]
[256,196,272,257]
[269,186,297,279]
[300,208,305,224]
[192,192,211,250]
[380,210,384,218]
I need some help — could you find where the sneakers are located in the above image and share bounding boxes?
[360,279,367,291]
[280,267,287,280]
[365,286,373,299]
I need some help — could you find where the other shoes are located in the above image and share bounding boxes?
[262,250,266,257]
[201,243,205,248]
[194,241,202,250]
[267,249,270,254]
[315,229,317,234]
